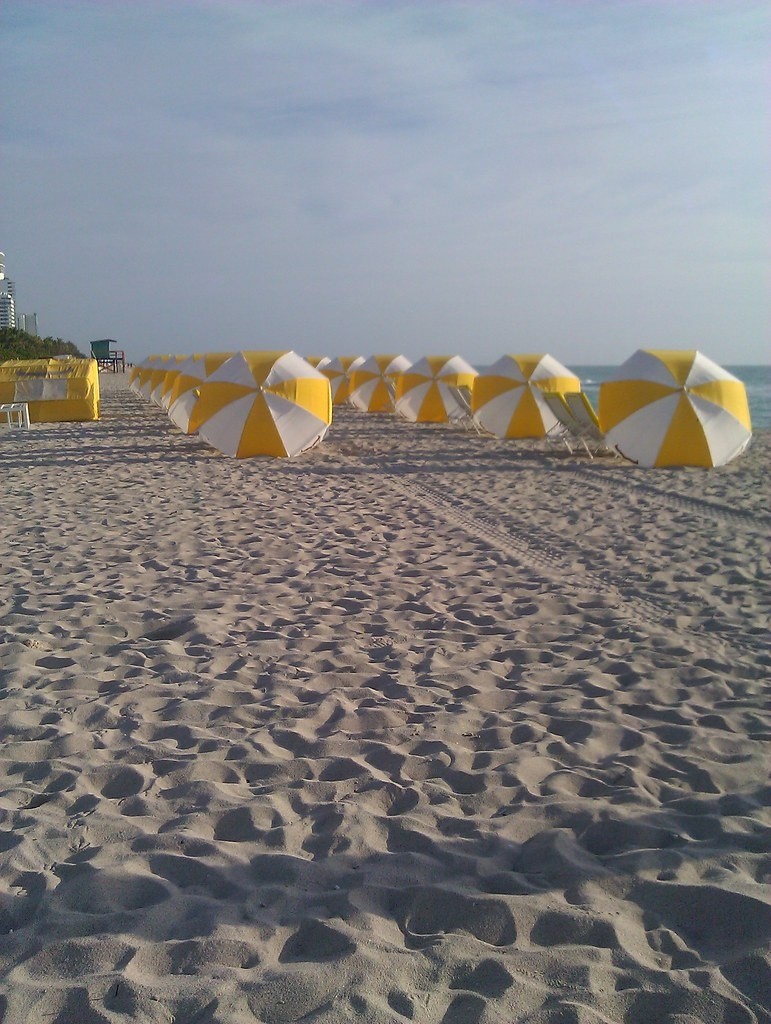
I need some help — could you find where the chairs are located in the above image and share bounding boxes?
[386,382,397,417]
[447,385,485,434]
[542,392,607,459]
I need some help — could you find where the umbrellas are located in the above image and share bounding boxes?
[323,357,365,404]
[473,354,580,439]
[168,353,231,434]
[199,350,331,456]
[599,349,752,468]
[304,357,331,371]
[397,355,479,424]
[129,354,202,412]
[350,354,412,412]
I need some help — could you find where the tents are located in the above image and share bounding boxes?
[0,358,100,423]
